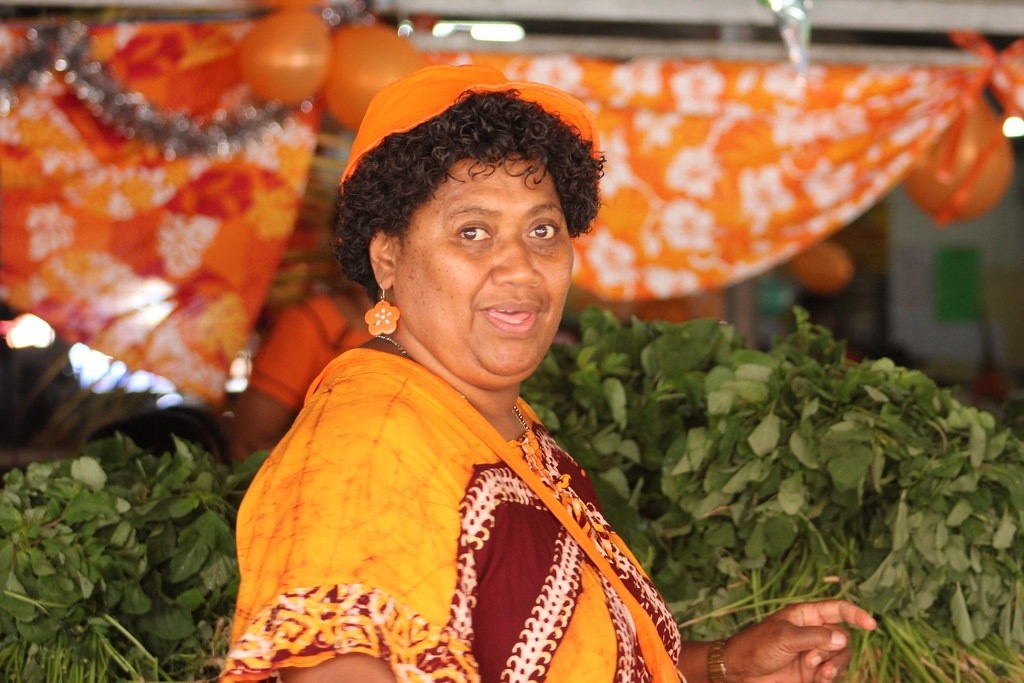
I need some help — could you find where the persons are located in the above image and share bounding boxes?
[223,286,373,470]
[219,62,876,683]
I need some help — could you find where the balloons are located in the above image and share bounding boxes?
[323,22,420,130]
[245,13,332,99]
[900,94,1015,222]
[792,240,856,294]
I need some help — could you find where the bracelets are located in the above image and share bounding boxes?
[708,640,729,683]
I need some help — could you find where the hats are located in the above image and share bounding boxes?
[338,65,599,222]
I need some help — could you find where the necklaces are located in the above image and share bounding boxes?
[375,334,530,430]
